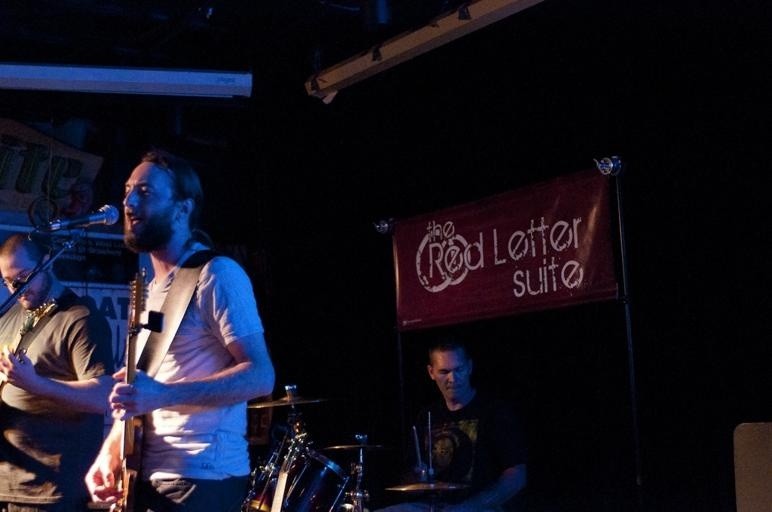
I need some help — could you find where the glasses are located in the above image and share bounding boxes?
[1,268,33,288]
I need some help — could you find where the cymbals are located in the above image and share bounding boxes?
[385,481,468,492]
[246,398,325,409]
[324,444,381,451]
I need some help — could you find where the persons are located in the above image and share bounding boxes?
[84,152,276,511]
[382,341,527,512]
[0,232,120,511]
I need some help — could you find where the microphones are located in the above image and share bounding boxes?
[49,204,119,232]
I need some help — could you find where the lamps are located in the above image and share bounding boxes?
[305,0,537,104]
[1,63,253,99]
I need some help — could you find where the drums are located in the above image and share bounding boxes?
[245,437,352,511]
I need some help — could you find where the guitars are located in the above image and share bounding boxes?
[0,300,57,389]
[112,273,150,511]
